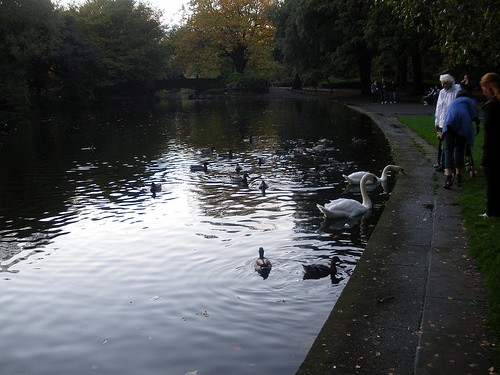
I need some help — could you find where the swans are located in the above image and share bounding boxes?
[316,173,378,219]
[341,165,408,185]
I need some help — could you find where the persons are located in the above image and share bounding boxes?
[433,68,462,168]
[420,87,434,105]
[389,79,398,104]
[441,89,481,188]
[478,73,500,218]
[371,79,378,104]
[379,77,388,104]
[460,73,470,88]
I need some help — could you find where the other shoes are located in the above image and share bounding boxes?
[480,213,488,218]
[437,165,444,172]
[433,164,440,167]
[456,174,462,186]
[443,175,453,189]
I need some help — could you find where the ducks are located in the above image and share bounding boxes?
[256,246,271,271]
[190,133,269,189]
[301,256,343,276]
[149,182,162,190]
